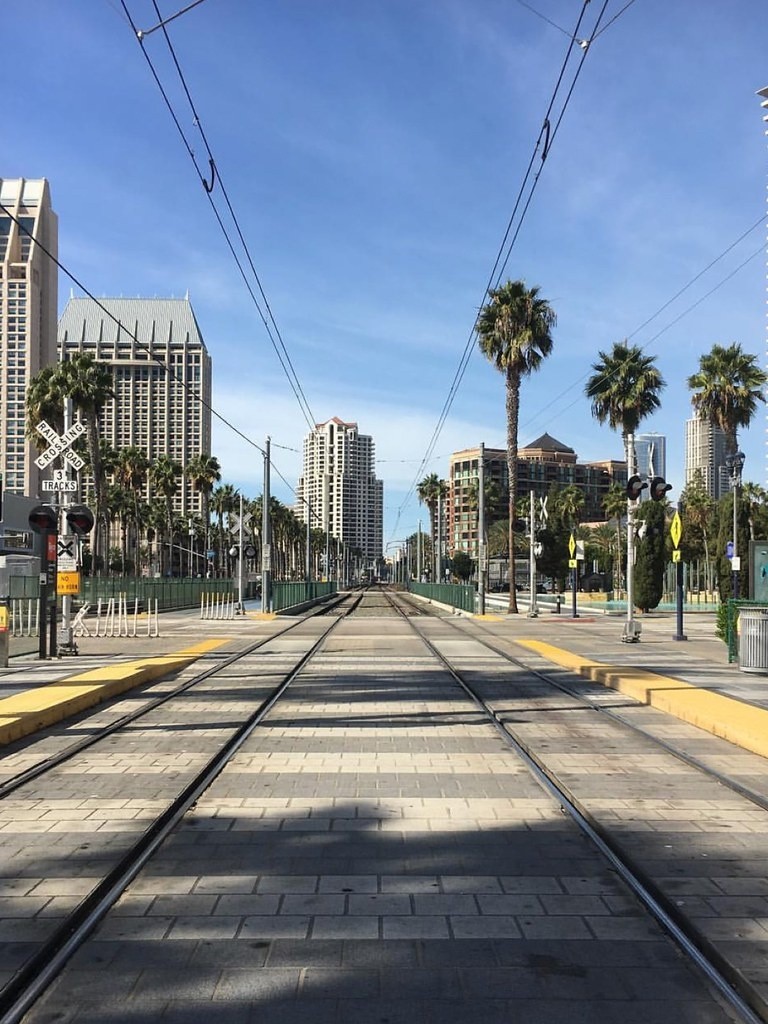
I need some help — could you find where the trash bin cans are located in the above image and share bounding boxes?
[736,605,768,673]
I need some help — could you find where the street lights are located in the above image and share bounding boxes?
[724,449,746,598]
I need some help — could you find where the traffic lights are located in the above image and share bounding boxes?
[512,520,527,533]
[66,507,94,535]
[29,505,58,536]
[628,476,648,502]
[650,477,673,504]
[536,528,553,543]
[229,544,257,559]
